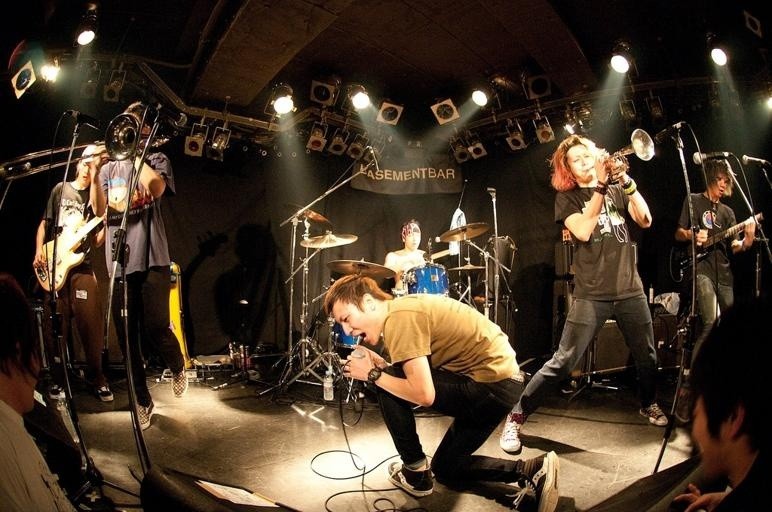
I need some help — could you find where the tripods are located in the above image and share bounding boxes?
[567,336,618,404]
[259,224,335,396]
[272,233,357,404]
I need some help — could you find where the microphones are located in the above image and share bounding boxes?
[435,237,440,242]
[66,110,105,133]
[345,336,364,403]
[369,146,380,172]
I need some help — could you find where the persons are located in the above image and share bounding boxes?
[34,144,115,402]
[90,103,188,431]
[0,274,78,512]
[673,160,756,422]
[384,219,433,292]
[671,297,772,512]
[324,274,559,512]
[500,135,669,453]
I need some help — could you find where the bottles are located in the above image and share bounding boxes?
[324,371,334,401]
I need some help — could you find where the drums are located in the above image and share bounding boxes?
[407,263,448,298]
[334,321,363,349]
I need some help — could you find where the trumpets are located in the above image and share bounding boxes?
[588,129,655,182]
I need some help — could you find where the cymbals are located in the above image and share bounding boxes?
[449,264,485,271]
[440,222,492,242]
[284,202,333,225]
[300,235,359,248]
[327,260,396,279]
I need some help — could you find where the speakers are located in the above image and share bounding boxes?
[139,463,301,512]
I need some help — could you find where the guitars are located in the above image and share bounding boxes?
[34,203,107,292]
[670,213,765,284]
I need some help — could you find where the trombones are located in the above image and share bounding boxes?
[0,113,179,180]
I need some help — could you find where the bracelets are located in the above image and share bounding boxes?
[594,181,608,195]
[621,175,637,196]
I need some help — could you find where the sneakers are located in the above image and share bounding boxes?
[49,385,66,399]
[675,393,691,423]
[98,386,113,402]
[171,364,188,397]
[134,401,155,431]
[500,412,528,452]
[639,402,668,426]
[385,462,434,497]
[505,451,559,512]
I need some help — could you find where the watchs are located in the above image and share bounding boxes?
[367,367,382,384]
